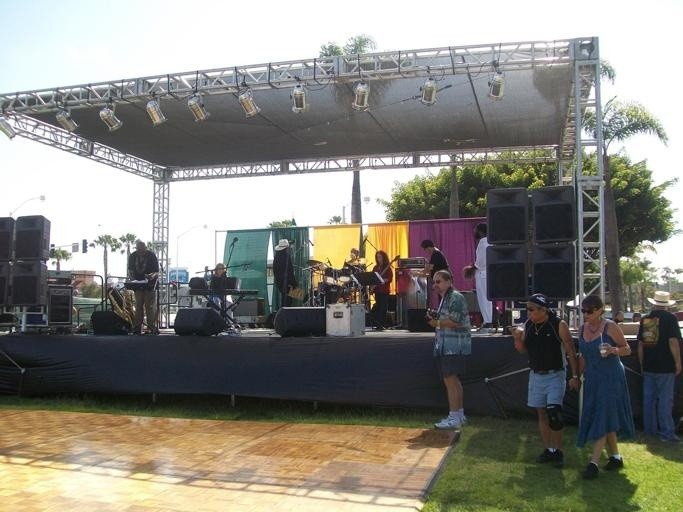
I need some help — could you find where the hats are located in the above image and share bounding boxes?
[274,239,295,252]
[647,291,676,307]
[528,294,550,308]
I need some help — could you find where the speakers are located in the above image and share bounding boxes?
[273,306,326,337]
[0,215,73,324]
[91,311,131,336]
[486,185,577,302]
[407,309,435,332]
[174,308,228,336]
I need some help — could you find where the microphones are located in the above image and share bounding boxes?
[327,260,332,266]
[288,240,294,243]
[359,238,366,248]
[230,237,238,247]
[308,240,313,246]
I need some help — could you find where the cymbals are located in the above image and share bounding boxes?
[306,259,328,271]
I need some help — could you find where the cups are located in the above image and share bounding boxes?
[513,323,526,337]
[598,344,609,358]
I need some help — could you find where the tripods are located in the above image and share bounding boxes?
[361,287,386,331]
[131,287,159,334]
[220,244,241,329]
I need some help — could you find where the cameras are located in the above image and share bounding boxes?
[423,310,440,321]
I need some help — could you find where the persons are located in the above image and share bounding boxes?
[420,239,453,306]
[613,310,625,324]
[568,293,636,481]
[425,268,472,430]
[203,262,234,333]
[368,250,394,329]
[127,241,159,335]
[462,221,494,328]
[272,238,298,307]
[511,292,580,468]
[345,247,372,311]
[635,290,682,444]
[632,311,641,322]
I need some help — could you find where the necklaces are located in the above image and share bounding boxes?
[588,322,603,337]
[534,318,547,338]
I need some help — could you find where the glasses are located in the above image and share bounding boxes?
[581,308,596,314]
[431,279,441,284]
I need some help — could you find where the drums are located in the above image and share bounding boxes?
[323,268,338,284]
[324,285,349,303]
[340,268,351,281]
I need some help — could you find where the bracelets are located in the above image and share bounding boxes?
[436,319,441,327]
[515,340,522,347]
[572,374,579,378]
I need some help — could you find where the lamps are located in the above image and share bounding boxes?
[289,84,308,114]
[0,115,16,140]
[486,69,506,102]
[419,78,439,107]
[99,104,123,133]
[237,87,261,119]
[351,80,370,112]
[55,108,79,133]
[145,99,168,128]
[187,93,210,124]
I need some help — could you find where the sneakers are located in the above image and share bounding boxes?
[535,448,624,480]
[660,434,682,444]
[434,416,468,429]
